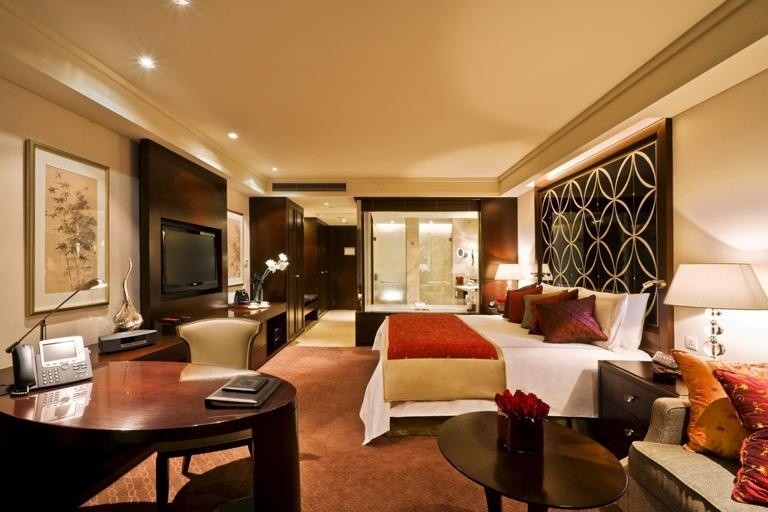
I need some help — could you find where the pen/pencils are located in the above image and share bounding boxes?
[163,317,182,321]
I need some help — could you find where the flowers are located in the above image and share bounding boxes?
[495,389,550,423]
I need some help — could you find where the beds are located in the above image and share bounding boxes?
[359,314,656,446]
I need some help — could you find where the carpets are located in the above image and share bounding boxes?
[74,347,654,512]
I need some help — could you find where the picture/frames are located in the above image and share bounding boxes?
[227,209,244,287]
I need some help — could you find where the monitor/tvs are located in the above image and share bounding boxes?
[161,224,219,293]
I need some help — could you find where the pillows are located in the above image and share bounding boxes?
[503,283,650,353]
[671,348,768,506]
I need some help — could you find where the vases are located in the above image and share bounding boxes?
[497,414,544,456]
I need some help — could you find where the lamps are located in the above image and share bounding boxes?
[662,262,768,360]
[494,264,531,291]
[541,263,554,284]
[6,276,109,353]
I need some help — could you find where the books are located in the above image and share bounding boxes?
[203,375,282,409]
[222,373,269,393]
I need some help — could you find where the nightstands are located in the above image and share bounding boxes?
[599,360,691,460]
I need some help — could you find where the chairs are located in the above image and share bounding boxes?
[157,318,262,505]
[620,398,768,512]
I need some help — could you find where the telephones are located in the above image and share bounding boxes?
[12,336,94,391]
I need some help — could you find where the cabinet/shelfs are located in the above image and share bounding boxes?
[26,139,111,317]
[84,301,289,369]
[249,197,329,340]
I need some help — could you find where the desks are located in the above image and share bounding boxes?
[0,361,301,512]
